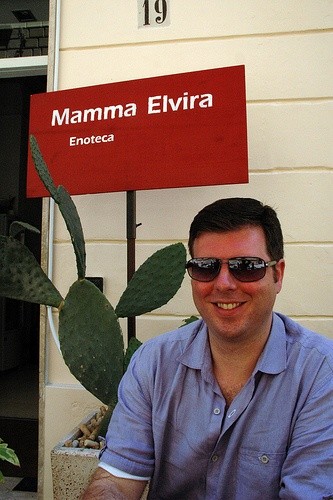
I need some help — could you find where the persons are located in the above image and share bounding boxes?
[79,198,333,500]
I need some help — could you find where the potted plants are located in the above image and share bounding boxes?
[1,135,190,500]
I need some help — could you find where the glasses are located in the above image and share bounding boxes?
[182,252,279,284]
